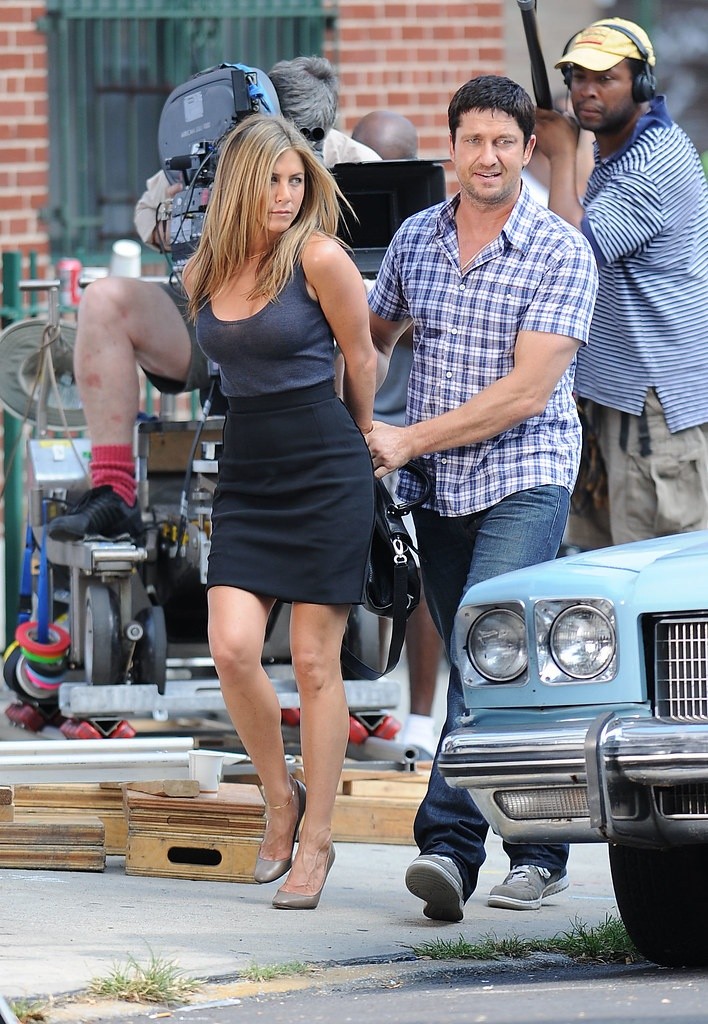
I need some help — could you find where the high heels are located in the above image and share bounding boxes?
[253,777,308,883]
[272,840,336,909]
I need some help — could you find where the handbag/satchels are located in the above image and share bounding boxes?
[340,455,432,682]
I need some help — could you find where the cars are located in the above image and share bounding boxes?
[436,521,708,960]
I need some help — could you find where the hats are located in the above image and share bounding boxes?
[554,17,656,71]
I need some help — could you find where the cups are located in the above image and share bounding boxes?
[187,750,225,799]
[109,239,143,279]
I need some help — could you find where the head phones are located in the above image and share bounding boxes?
[561,24,656,104]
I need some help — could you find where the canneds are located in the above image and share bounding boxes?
[56,258,84,306]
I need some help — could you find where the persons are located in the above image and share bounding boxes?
[49,56,382,547]
[355,111,445,753]
[179,114,377,910]
[530,17,708,549]
[363,76,601,923]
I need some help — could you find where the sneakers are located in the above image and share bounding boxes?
[48,484,149,547]
[487,863,570,909]
[405,853,465,922]
